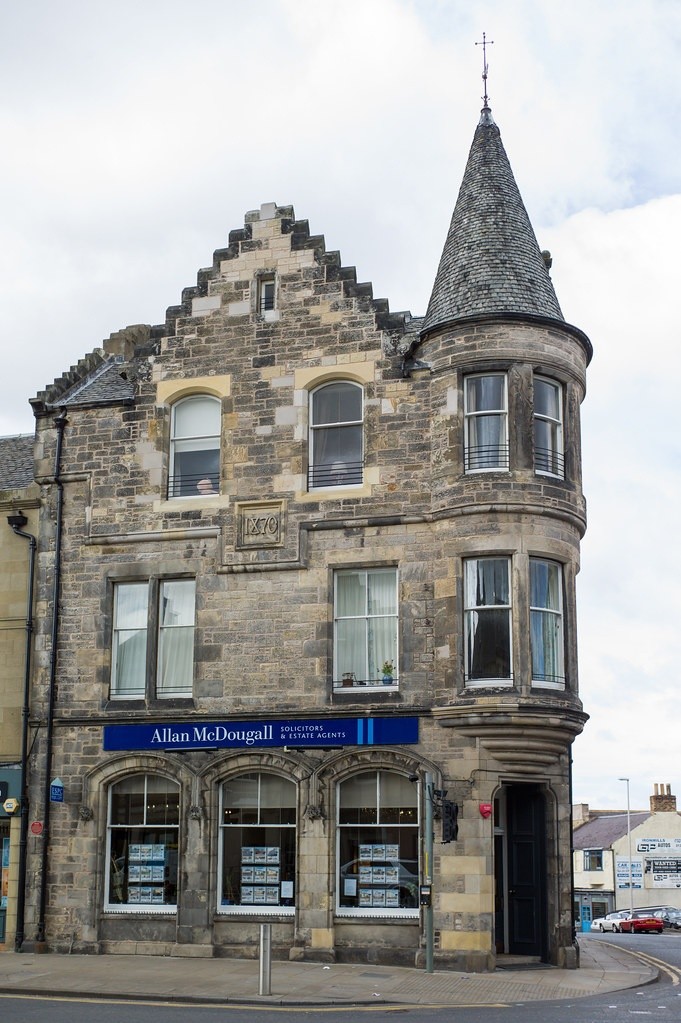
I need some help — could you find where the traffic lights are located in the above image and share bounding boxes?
[442,801,460,842]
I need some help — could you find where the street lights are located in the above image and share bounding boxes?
[619,777,633,915]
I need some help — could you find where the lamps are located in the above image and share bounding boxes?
[330,460,347,485]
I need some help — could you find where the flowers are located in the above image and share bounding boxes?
[377,659,395,675]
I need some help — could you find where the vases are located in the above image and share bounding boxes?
[382,675,393,684]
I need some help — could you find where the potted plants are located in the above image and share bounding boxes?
[342,672,354,686]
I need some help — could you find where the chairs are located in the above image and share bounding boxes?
[197,479,216,494]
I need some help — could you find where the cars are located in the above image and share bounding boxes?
[600,912,640,933]
[619,913,663,934]
